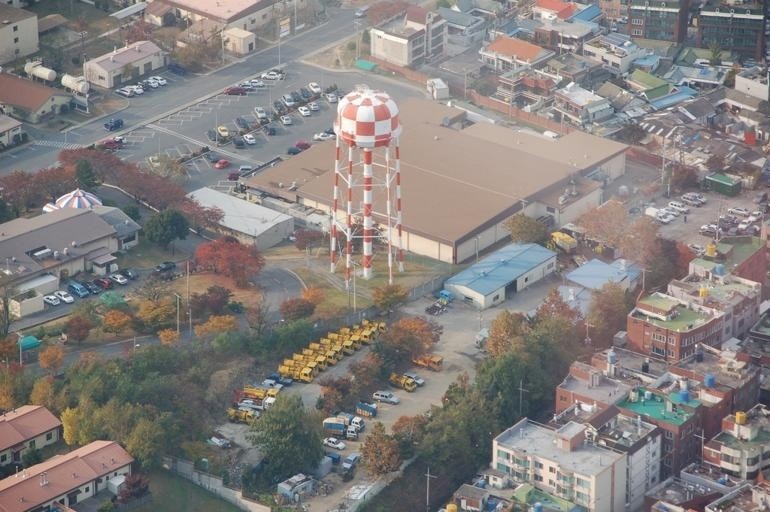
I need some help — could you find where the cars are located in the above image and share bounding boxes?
[655,192,769,259]
[115,75,167,98]
[44,261,177,306]
[97,136,126,150]
[206,71,338,180]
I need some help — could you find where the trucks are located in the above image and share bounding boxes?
[103,118,122,131]
[226,318,443,482]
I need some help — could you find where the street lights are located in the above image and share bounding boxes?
[77,31,90,112]
[16,332,24,368]
[174,292,181,333]
